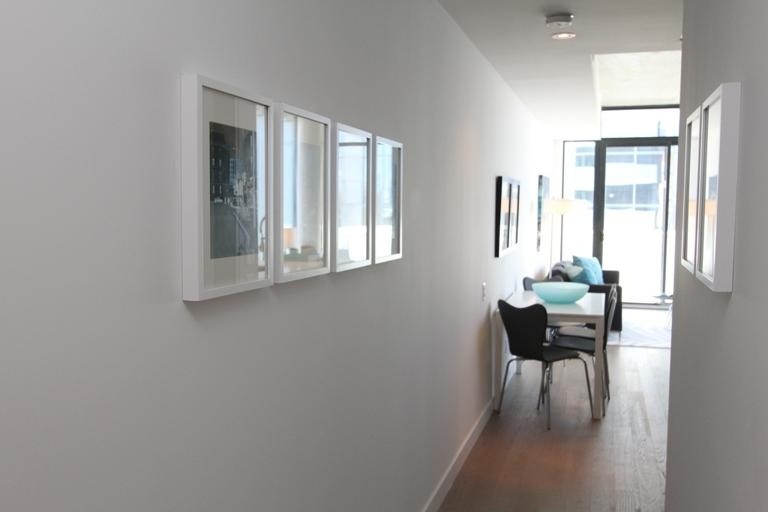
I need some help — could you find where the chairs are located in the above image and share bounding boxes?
[497,277,617,429]
[552,259,623,332]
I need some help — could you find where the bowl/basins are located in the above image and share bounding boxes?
[531,280,591,305]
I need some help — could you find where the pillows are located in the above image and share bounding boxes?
[567,256,604,284]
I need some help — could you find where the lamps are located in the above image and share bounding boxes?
[547,14,578,41]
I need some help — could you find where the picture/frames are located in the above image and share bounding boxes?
[181,74,403,302]
[678,79,741,296]
[496,175,522,257]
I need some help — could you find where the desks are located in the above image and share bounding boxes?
[493,289,605,419]
